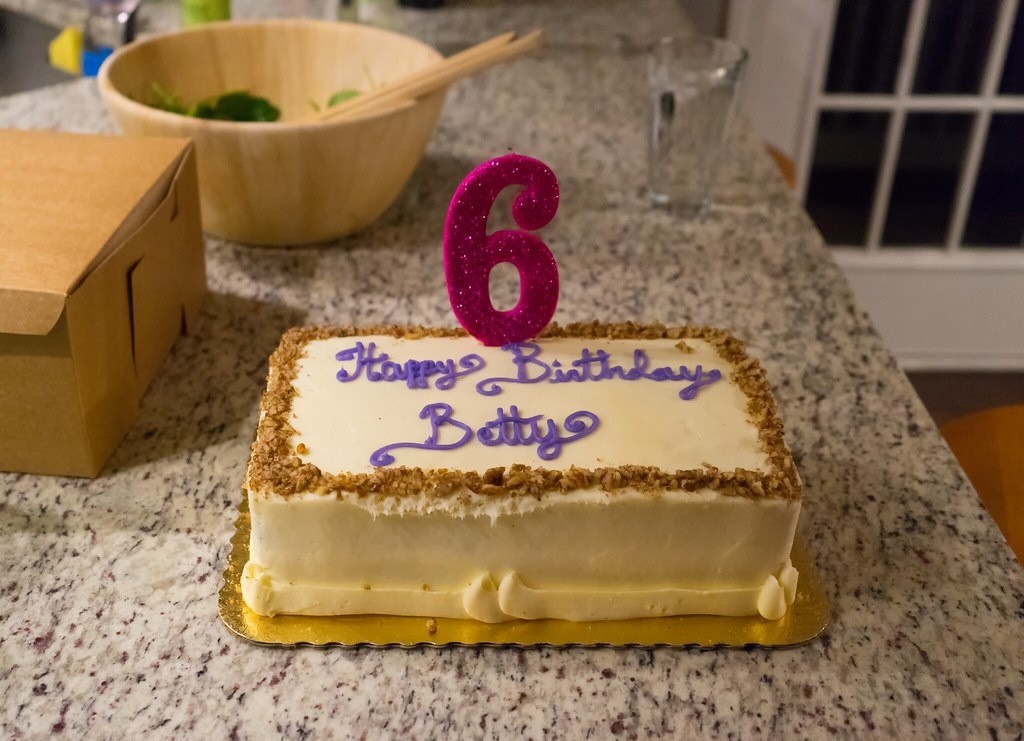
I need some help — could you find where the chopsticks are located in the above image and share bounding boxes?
[308,30,543,124]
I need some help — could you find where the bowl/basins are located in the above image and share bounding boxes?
[96,21,446,244]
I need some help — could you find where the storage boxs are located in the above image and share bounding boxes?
[0,127,208,480]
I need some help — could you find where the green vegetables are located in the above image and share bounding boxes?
[145,82,362,120]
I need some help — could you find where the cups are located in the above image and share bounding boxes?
[641,37,747,210]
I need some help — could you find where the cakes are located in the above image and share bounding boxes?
[237,319,802,620]
